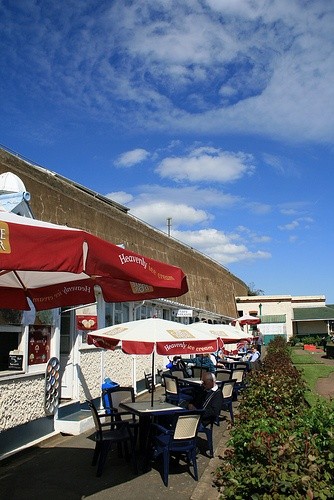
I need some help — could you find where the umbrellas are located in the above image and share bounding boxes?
[187,321,257,380]
[87,315,224,407]
[0,212,189,311]
[232,312,261,333]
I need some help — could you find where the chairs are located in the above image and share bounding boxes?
[85,348,259,486]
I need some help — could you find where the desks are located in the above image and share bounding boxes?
[178,377,222,406]
[118,401,185,458]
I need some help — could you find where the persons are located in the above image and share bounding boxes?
[252,329,264,345]
[159,373,218,435]
[248,345,261,362]
[182,354,216,374]
